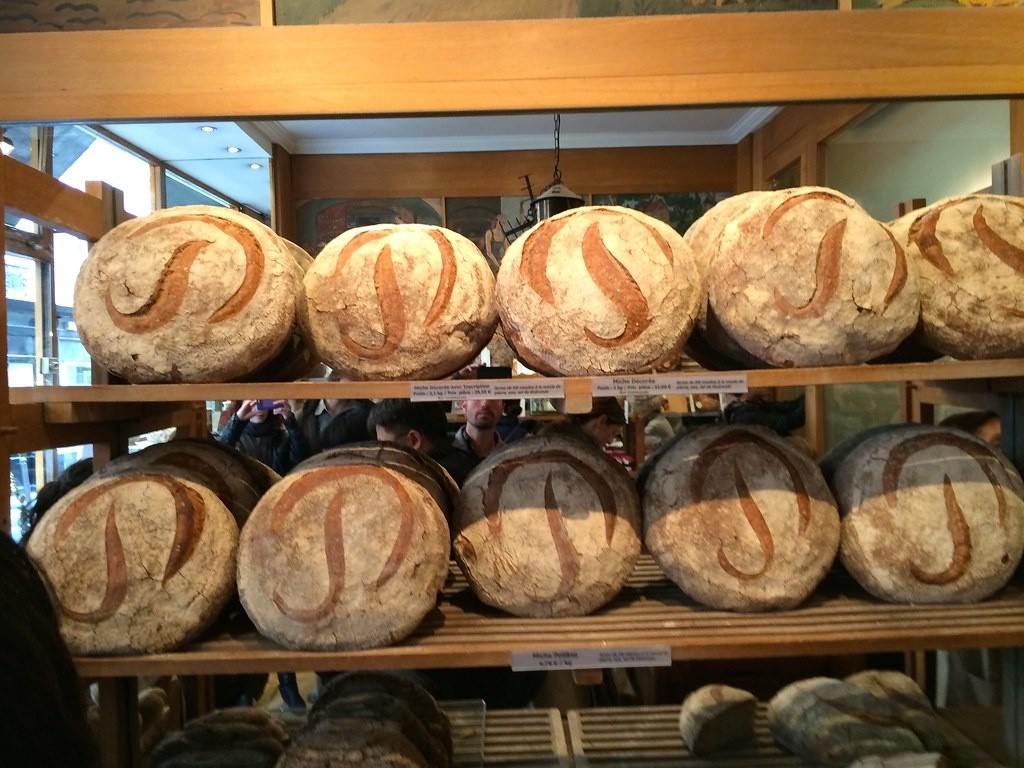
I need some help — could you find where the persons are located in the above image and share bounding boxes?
[218,361,626,490]
[722,387,806,438]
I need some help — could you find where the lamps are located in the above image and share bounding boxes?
[530,114,584,223]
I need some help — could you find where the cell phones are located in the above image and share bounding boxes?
[477,366,512,379]
[256,399,284,410]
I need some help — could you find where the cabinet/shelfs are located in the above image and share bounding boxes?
[0,6,1024,768]
